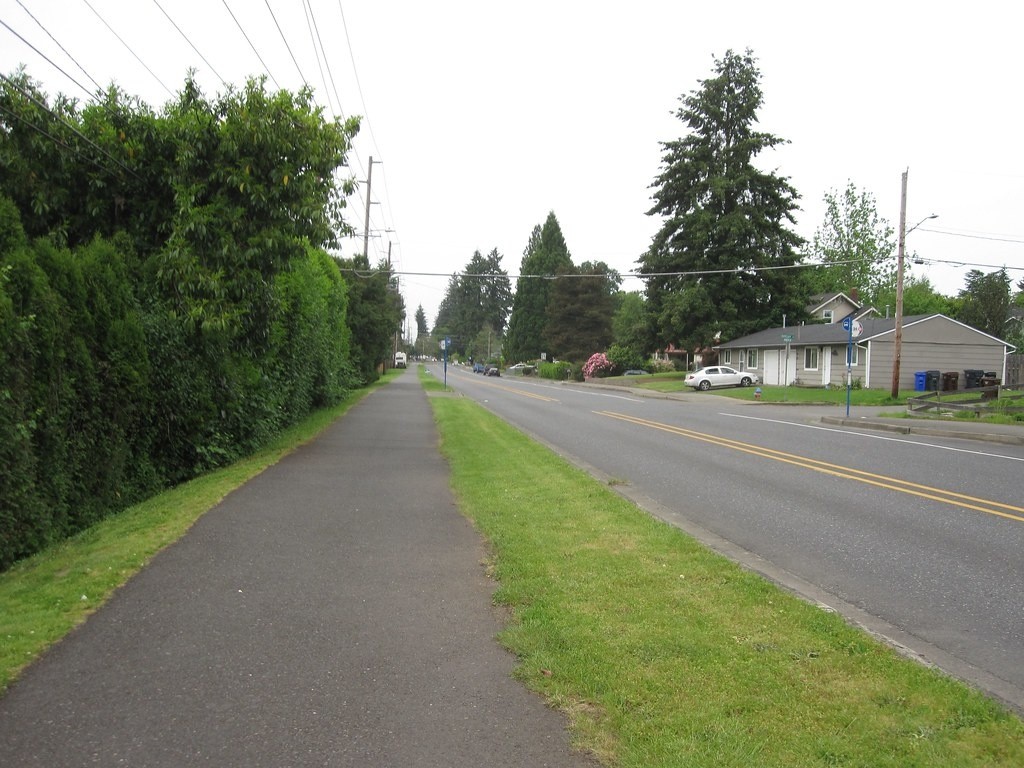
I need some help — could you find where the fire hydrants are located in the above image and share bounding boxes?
[754,386,762,401]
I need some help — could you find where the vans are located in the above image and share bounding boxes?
[483,364,497,375]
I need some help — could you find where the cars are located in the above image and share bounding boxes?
[621,370,650,377]
[683,365,759,391]
[488,367,501,377]
[472,363,484,373]
[509,363,534,371]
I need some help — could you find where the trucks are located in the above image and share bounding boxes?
[394,352,407,367]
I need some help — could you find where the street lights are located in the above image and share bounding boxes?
[890,213,939,399]
[363,228,395,260]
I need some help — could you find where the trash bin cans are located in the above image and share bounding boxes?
[926,370,940,391]
[942,372,959,391]
[980,378,1002,399]
[964,369,997,389]
[915,372,926,391]
